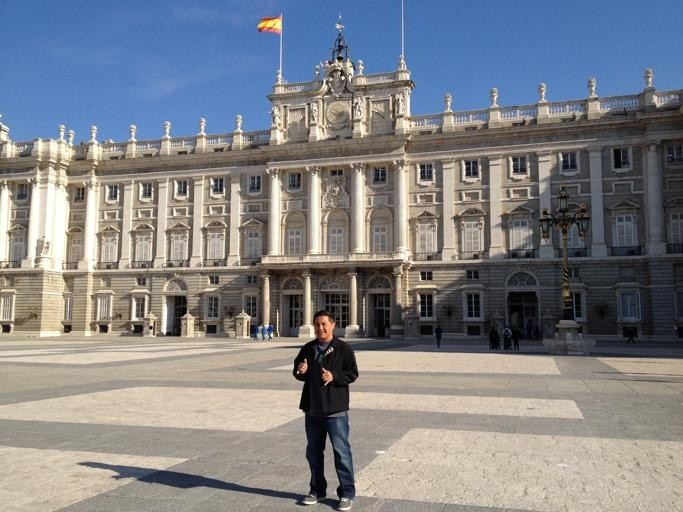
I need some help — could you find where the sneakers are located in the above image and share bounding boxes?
[302,491,328,505]
[338,496,353,510]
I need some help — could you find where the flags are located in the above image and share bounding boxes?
[257,15,281,35]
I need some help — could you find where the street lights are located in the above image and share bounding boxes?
[534,179,593,357]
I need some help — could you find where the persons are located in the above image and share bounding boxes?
[624,326,636,344]
[260,325,266,340]
[487,324,520,350]
[266,324,273,340]
[434,324,443,348]
[253,324,258,340]
[292,310,356,512]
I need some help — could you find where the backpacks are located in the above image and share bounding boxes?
[504,330,509,336]
